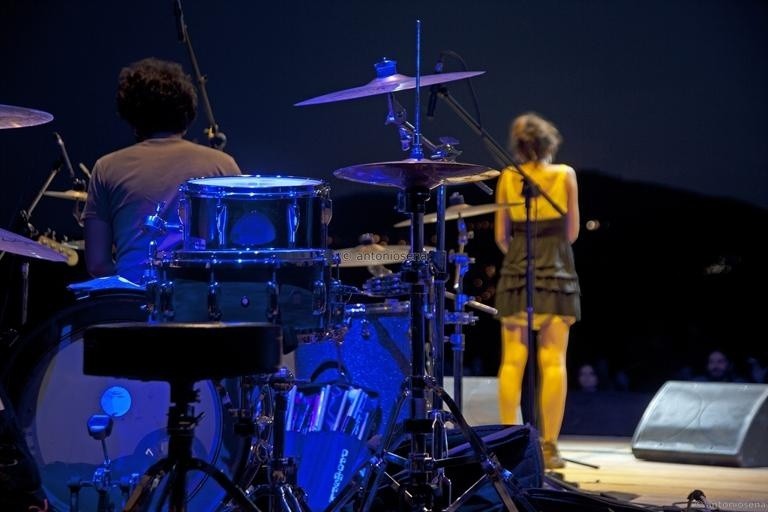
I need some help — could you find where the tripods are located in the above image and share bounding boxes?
[323,173,544,512]
[217,370,305,512]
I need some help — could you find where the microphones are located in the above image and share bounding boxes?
[53,132,75,178]
[426,52,447,117]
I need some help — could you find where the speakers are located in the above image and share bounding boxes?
[632,380,768,467]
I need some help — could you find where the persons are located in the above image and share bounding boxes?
[493,113,583,470]
[691,350,750,383]
[77,57,243,283]
[747,357,768,383]
[614,368,639,392]
[578,363,601,392]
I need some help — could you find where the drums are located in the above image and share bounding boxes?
[144,249,333,345]
[295,302,411,432]
[180,172,329,250]
[3,292,280,511]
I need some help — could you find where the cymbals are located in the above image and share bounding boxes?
[394,202,523,227]
[333,158,501,188]
[44,191,89,201]
[293,70,485,107]
[0,228,68,262]
[0,105,54,129]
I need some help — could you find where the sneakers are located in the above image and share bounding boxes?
[538,436,565,469]
